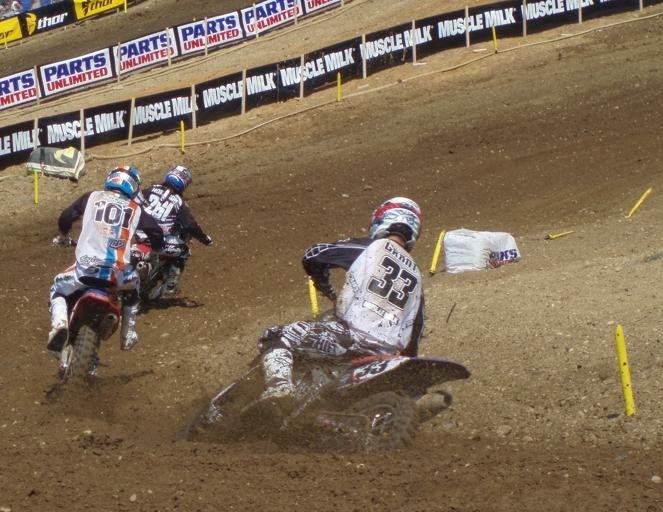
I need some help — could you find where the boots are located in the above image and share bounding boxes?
[119,304,139,351]
[45,297,69,353]
[240,348,298,414]
[164,274,181,295]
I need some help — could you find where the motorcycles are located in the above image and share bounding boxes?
[47,235,155,399]
[176,277,476,459]
[130,224,192,314]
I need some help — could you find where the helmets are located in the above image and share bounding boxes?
[368,196,423,248]
[104,165,143,198]
[165,165,193,192]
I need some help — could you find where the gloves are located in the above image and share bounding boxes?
[144,248,160,264]
[59,231,72,248]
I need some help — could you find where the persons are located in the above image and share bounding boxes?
[239,196,426,420]
[46,166,163,350]
[138,166,211,293]
[0,0,62,22]
